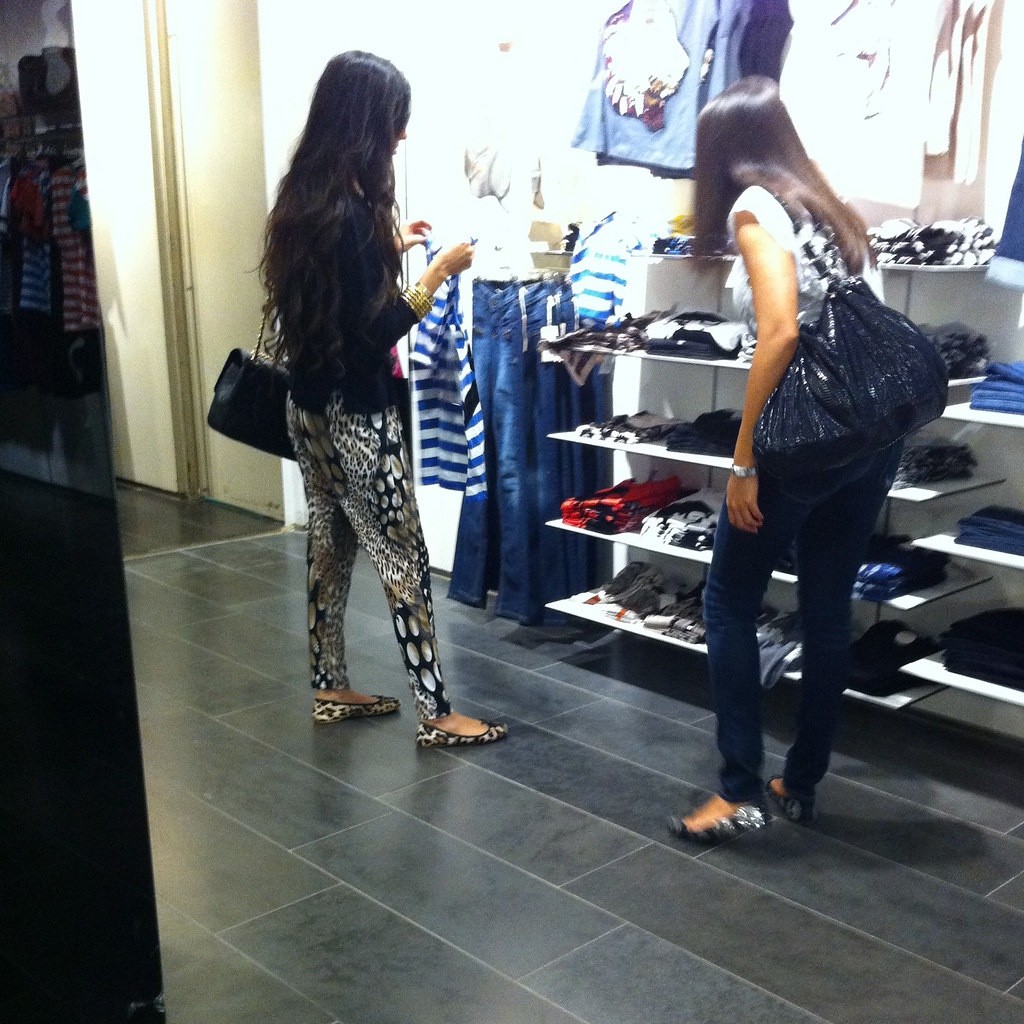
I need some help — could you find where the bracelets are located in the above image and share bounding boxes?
[401,281,436,320]
[731,464,757,477]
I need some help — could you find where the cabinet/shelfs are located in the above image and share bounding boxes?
[545,249,1024,712]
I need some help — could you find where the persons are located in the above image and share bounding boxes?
[260,52,508,749]
[670,75,905,839]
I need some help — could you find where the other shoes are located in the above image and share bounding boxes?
[416,719,509,748]
[763,773,814,822]
[312,695,401,724]
[667,796,769,838]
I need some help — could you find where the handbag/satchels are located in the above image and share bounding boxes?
[752,184,948,478]
[207,292,298,460]
